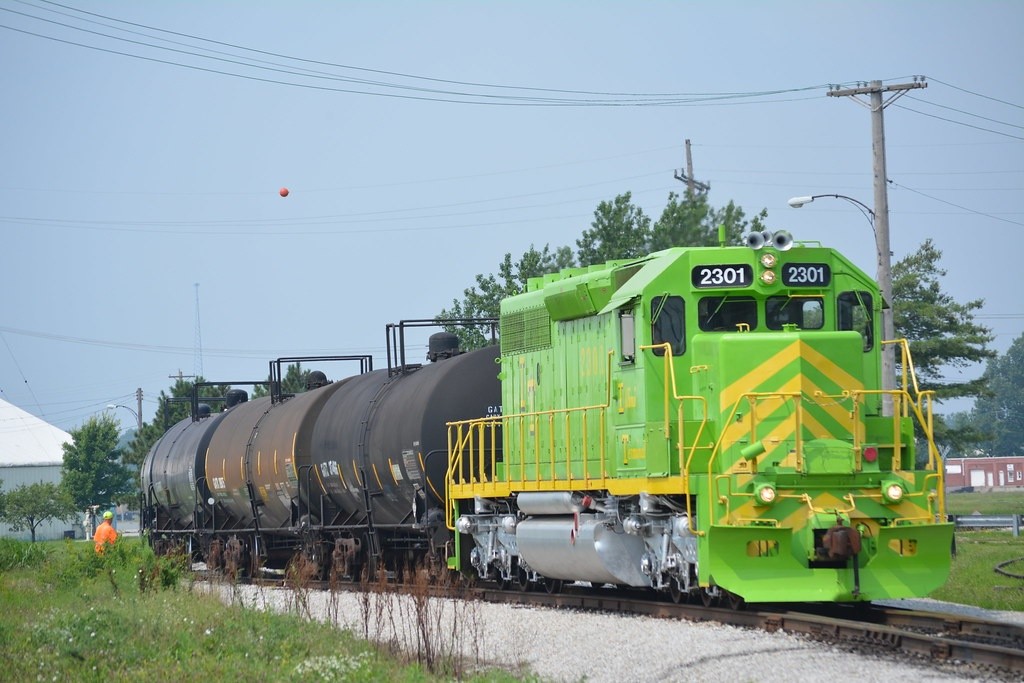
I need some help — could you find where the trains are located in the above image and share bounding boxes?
[141,227,958,609]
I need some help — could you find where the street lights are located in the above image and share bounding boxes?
[788,193,900,417]
[108,404,143,436]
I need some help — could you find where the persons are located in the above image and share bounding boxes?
[94,511,118,555]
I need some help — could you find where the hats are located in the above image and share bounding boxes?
[103,511,114,519]
